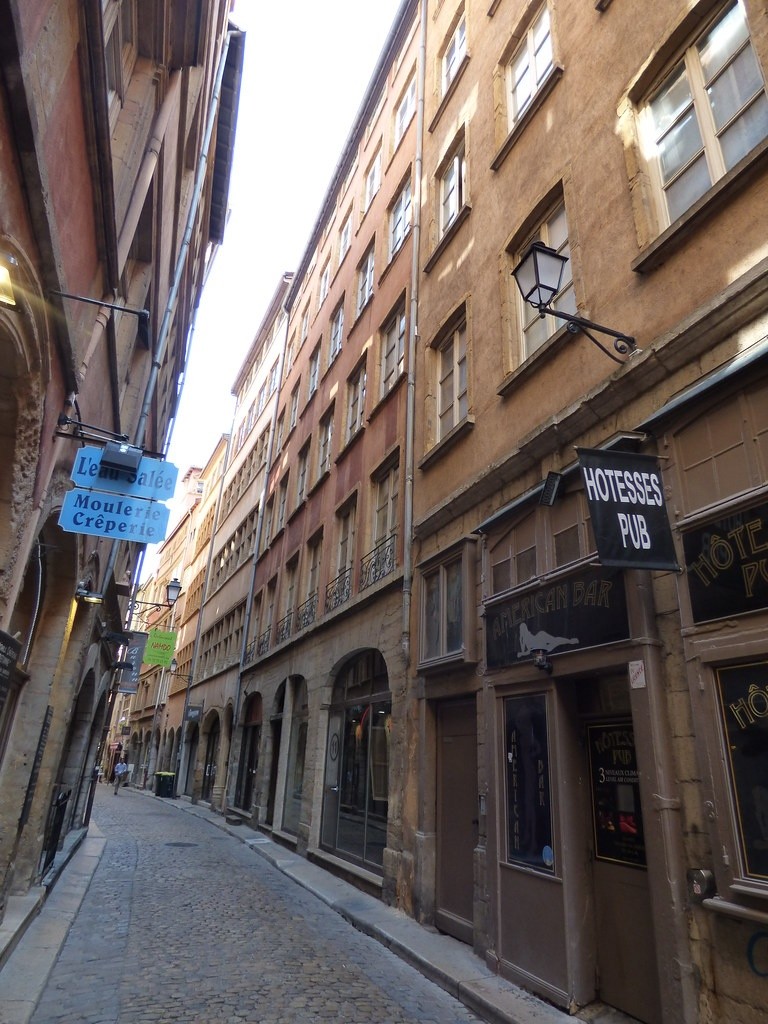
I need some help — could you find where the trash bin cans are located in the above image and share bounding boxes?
[154,771,175,798]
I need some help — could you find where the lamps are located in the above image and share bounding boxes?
[112,661,134,671]
[127,577,182,623]
[106,630,130,648]
[170,658,194,687]
[75,588,104,606]
[510,241,637,366]
[540,471,566,507]
[530,648,553,672]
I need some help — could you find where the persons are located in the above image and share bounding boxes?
[114,758,127,795]
[99,766,103,782]
